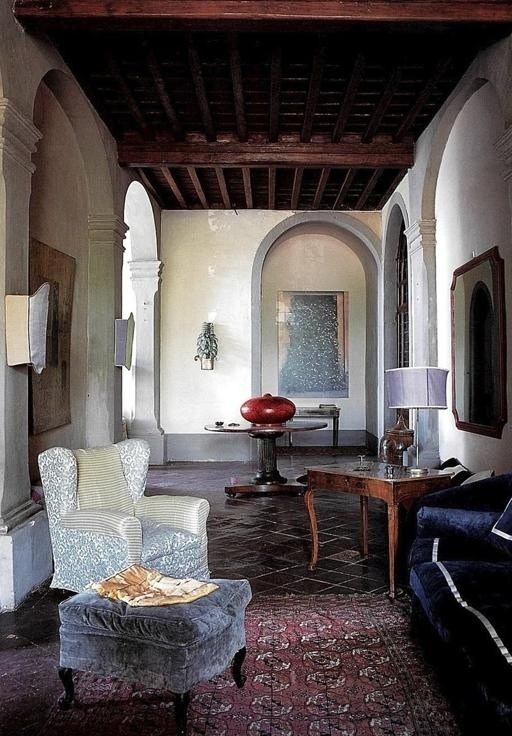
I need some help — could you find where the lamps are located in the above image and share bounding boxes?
[383,366,449,469]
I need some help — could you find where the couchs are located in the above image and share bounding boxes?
[409,472,511,736]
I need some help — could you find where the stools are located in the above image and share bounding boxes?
[57,563,253,736]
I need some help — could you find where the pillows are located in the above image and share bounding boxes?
[484,497,512,556]
[416,506,501,548]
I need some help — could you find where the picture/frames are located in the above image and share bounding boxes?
[450,245,508,439]
[276,290,349,399]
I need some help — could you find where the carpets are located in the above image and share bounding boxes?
[42,592,475,736]
[276,445,373,457]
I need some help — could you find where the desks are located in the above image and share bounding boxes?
[288,406,340,447]
[304,460,452,599]
[203,420,328,498]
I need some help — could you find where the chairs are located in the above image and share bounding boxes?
[38,438,211,596]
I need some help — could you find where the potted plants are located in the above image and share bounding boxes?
[193,321,219,371]
